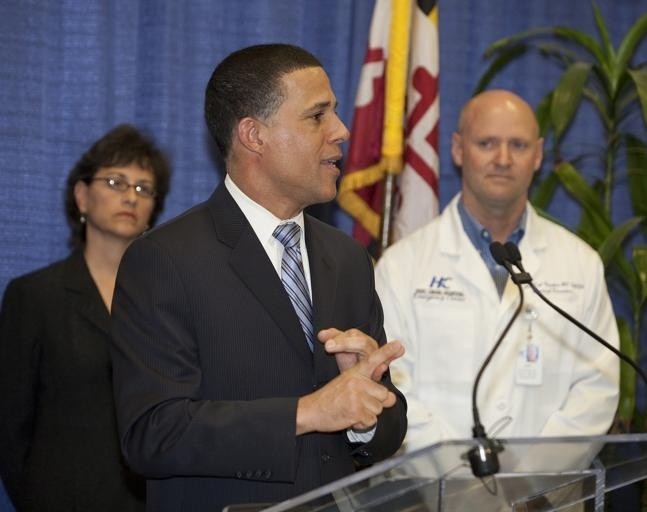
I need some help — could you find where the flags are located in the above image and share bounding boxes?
[333,0,440,262]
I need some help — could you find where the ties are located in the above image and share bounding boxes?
[273,223,315,351]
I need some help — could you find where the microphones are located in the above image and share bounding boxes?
[469,240,523,475]
[502,241,647,383]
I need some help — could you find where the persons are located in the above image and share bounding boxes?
[373,87,622,512]
[108,41,408,511]
[526,347,537,363]
[1,122,169,512]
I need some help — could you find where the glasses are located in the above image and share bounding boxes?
[94,175,158,198]
